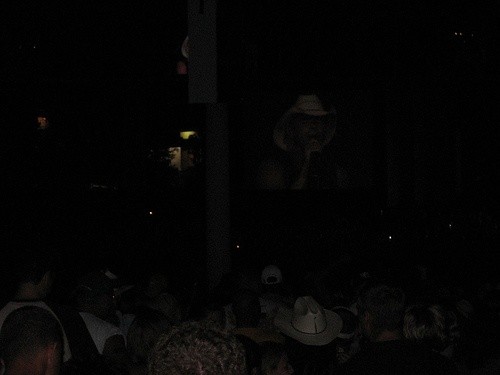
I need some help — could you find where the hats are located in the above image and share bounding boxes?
[262,265,282,284]
[273,95,336,152]
[274,296,342,346]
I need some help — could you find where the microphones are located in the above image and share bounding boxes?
[309,138,320,167]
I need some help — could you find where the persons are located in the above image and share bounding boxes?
[268,94,340,192]
[0,256,500,375]
